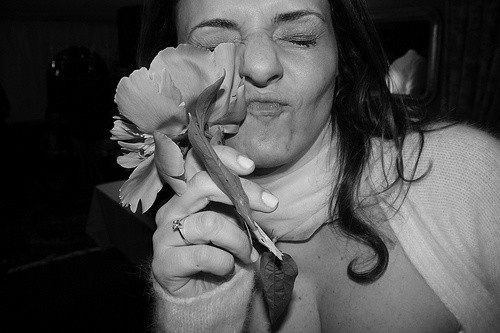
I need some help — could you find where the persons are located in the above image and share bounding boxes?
[135,1,500,333]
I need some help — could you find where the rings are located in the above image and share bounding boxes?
[173,215,196,245]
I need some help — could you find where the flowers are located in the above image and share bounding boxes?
[107,40,300,333]
